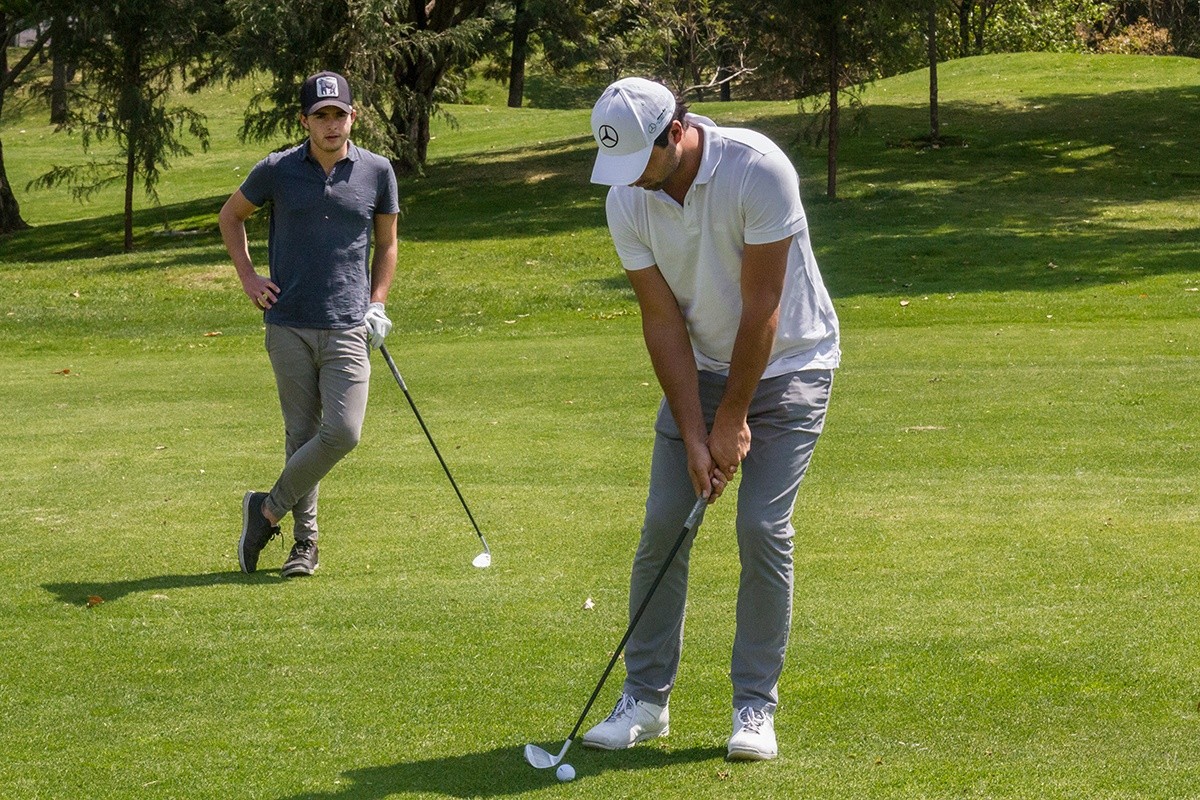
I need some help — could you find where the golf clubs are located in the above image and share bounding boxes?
[369,322,493,568]
[522,481,717,770]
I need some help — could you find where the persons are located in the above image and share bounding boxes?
[581,77,841,762]
[218,72,401,578]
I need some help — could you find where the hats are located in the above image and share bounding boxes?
[301,71,352,115]
[590,77,675,185]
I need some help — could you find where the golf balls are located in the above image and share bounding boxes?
[555,764,576,783]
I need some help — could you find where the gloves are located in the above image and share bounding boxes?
[364,302,392,351]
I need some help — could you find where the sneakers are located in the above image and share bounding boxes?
[282,539,319,577]
[238,490,284,575]
[582,690,670,749]
[727,706,777,760]
